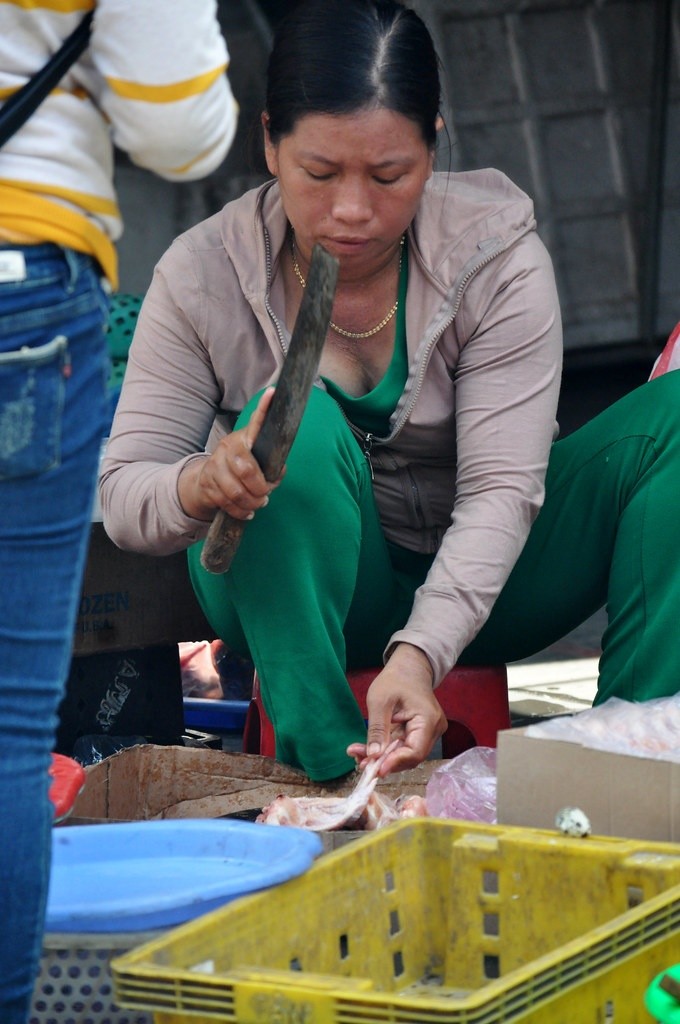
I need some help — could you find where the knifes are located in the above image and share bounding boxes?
[199,242,342,573]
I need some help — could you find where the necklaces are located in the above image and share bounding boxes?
[292,235,404,337]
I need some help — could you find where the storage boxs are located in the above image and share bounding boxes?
[104,810,679,1023]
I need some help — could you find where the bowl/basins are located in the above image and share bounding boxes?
[43,753,319,932]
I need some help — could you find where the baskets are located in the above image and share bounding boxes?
[28,930,159,1024]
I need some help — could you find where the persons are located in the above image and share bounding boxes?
[101,0,680,776]
[0,0,238,1023]
